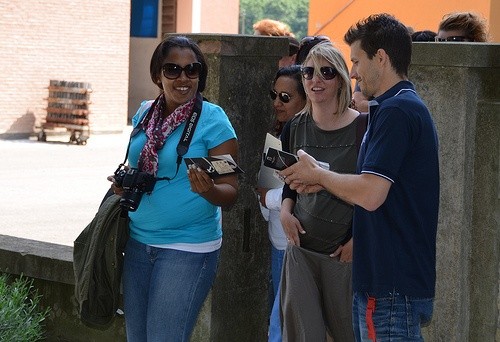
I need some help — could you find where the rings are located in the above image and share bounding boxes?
[287,238,291,242]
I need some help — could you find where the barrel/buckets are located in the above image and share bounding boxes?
[47,79,90,126]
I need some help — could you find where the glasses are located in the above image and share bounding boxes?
[270,90,303,103]
[301,66,338,80]
[160,63,202,79]
[434,36,471,42]
[302,36,330,44]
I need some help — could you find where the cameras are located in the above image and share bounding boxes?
[114,168,155,212]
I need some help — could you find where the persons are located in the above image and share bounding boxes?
[435,11,490,42]
[411,30,438,42]
[280,40,354,342]
[289,34,300,66]
[295,35,334,65]
[252,19,294,37]
[353,82,368,112]
[279,13,440,342]
[254,65,307,342]
[107,37,241,342]
